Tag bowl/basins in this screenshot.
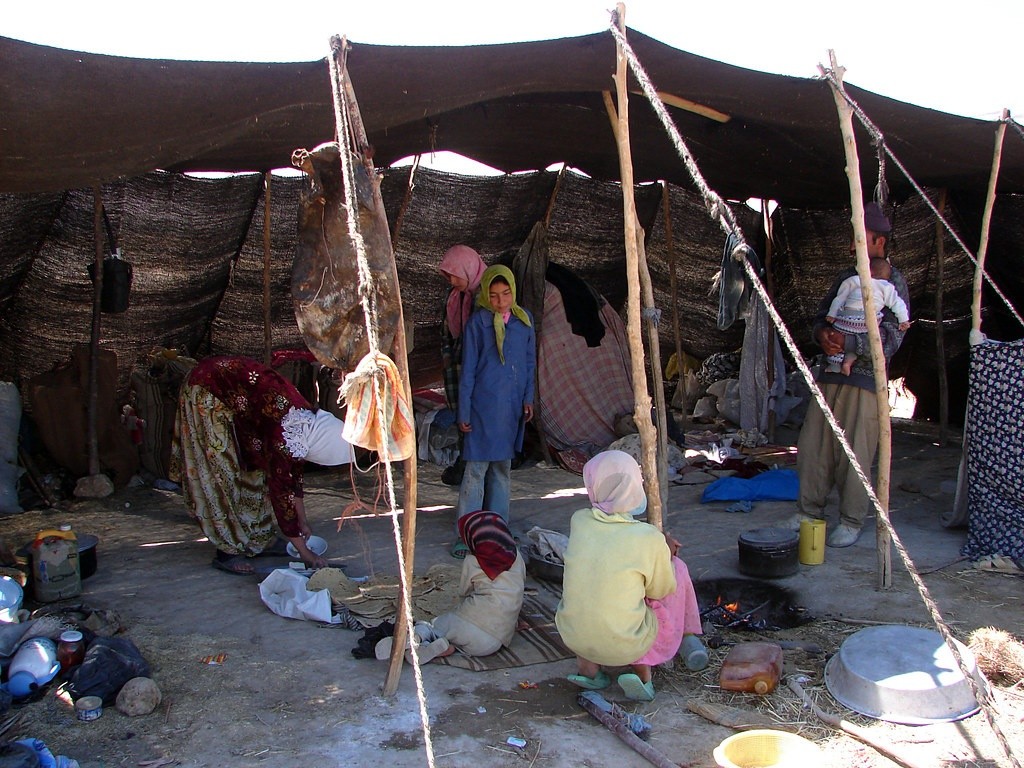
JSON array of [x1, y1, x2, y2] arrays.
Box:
[[824, 625, 991, 725]]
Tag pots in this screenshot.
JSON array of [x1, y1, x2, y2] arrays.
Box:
[[738, 528, 801, 578], [16, 532, 99, 581], [713, 730, 826, 768]]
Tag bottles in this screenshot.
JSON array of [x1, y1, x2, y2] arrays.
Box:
[[57, 631, 85, 682], [680, 634, 709, 671], [32, 525, 82, 601], [720, 642, 783, 694]]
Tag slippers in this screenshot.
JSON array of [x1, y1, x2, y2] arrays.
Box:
[[567, 670, 613, 690], [213, 550, 258, 577], [972, 552, 1024, 576], [617, 671, 656, 702], [449, 539, 471, 559], [258, 536, 290, 557]]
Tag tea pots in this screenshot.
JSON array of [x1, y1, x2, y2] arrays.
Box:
[[0, 637, 62, 696]]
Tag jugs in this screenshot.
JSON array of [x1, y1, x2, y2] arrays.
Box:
[[800, 519, 826, 564]]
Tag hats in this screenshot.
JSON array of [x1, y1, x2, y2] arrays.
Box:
[[862, 200, 892, 233]]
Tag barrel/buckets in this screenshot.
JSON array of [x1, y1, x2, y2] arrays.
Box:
[[0, 576, 23, 626]]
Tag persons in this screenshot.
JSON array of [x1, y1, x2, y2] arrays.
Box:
[[772, 200, 909, 548], [375, 510, 526, 667], [438, 245, 537, 559], [168, 355, 356, 578], [554, 449, 702, 701], [825, 258, 909, 376]]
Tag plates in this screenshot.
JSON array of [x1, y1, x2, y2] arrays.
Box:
[[287, 536, 328, 558]]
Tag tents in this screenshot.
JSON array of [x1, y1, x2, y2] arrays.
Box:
[[0, 0, 1024, 692]]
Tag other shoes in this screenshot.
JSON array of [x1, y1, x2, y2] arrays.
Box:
[[404, 636, 450, 667], [375, 631, 420, 661], [826, 523, 863, 548], [771, 513, 817, 534]]
